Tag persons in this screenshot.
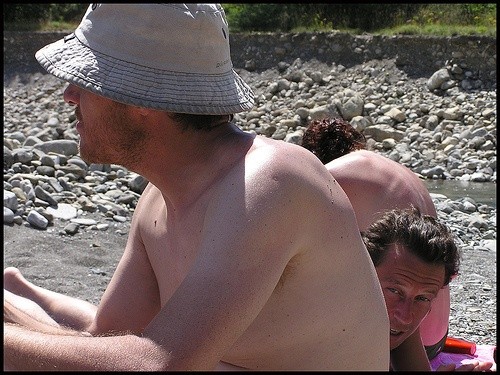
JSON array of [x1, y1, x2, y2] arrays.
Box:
[[301, 119, 450, 361], [3, 209, 493, 372], [3, 4, 390, 371]]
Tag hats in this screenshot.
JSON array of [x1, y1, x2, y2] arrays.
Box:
[[34, 4, 255, 115]]
